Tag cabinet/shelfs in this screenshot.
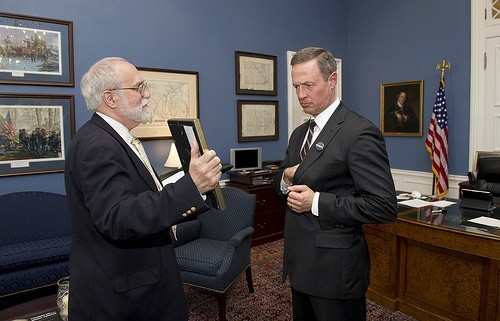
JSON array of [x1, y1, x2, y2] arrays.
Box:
[[223, 180, 284, 247]]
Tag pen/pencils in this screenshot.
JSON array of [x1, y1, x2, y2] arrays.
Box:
[[431, 210, 447, 213]]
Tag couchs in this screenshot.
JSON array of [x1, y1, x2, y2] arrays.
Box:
[[0, 191, 72, 301]]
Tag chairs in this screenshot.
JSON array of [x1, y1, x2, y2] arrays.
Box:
[[459, 154, 500, 209], [174, 187, 257, 321]]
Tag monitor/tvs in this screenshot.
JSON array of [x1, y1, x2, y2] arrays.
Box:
[[230, 147, 262, 173]]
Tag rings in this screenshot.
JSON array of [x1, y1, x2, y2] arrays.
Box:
[[291, 203, 293, 209]]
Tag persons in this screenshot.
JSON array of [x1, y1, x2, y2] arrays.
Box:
[[64, 57, 222, 321], [274, 46, 397, 321], [388, 89, 419, 133], [14, 43, 51, 62], [0, 127, 59, 156]]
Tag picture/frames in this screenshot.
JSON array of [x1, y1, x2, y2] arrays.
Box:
[[237, 100, 279, 143], [0, 93, 76, 175], [129, 67, 199, 140], [286, 50, 342, 143], [235, 50, 278, 96], [379, 80, 423, 137], [0, 12, 76, 87]]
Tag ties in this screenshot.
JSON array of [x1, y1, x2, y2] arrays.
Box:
[[131, 137, 177, 240], [301, 120, 315, 162]]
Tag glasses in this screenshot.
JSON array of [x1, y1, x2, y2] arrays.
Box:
[[109, 80, 148, 97]]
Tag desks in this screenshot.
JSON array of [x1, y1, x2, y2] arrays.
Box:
[[360, 192, 500, 321]]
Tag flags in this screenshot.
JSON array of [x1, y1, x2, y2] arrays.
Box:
[[425, 76, 449, 197], [3, 117, 14, 137], [424, 197, 446, 226]]
